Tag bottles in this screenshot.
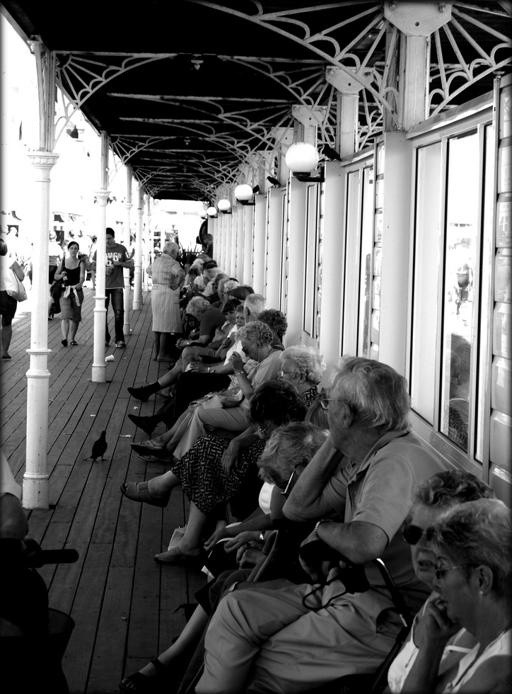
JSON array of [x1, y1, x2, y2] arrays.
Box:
[[62, 274, 69, 287]]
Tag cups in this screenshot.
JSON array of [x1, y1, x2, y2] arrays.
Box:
[[105, 355, 115, 362]]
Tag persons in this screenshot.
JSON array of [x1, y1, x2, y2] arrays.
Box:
[[50, 229, 97, 347], [369, 470, 512, 694], [176, 252, 254, 352], [92, 227, 134, 347], [0, 445, 29, 542], [146, 242, 185, 362], [195, 357, 446, 694], [0, 238, 24, 359], [119, 293, 329, 694]]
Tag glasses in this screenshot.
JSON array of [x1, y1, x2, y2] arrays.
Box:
[[401, 524, 437, 544]]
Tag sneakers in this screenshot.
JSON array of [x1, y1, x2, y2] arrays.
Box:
[[122, 384, 198, 694]]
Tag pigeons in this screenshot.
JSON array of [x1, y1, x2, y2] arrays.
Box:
[[90, 430, 108, 462]]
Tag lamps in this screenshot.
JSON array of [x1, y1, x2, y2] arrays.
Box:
[[285, 142, 325, 182], [235, 184, 255, 205], [207, 207, 218, 218], [218, 199, 232, 214]]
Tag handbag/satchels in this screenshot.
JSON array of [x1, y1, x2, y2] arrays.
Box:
[[4, 269, 27, 301], [50, 278, 62, 299]]
